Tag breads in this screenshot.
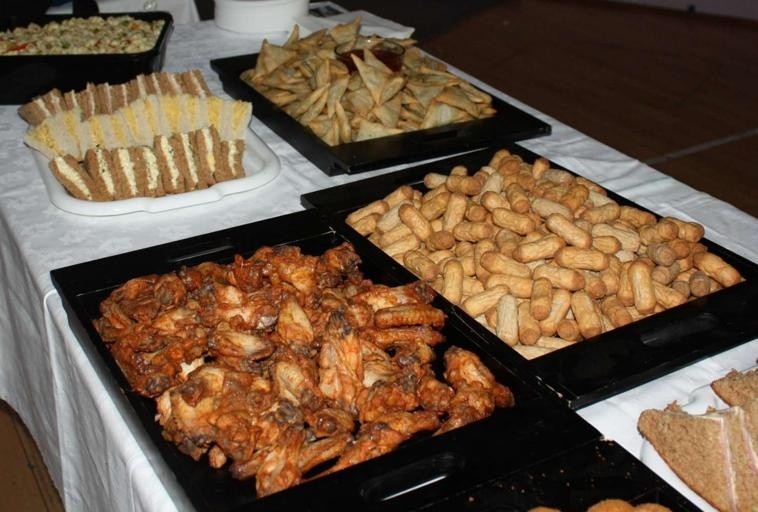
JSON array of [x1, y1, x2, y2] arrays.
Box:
[[17, 68, 253, 201], [638, 368, 758, 511]]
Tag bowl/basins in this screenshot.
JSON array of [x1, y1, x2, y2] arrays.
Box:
[[334, 41, 406, 74]]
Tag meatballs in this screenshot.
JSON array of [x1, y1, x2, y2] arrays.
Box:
[[346, 149, 741, 359]]
